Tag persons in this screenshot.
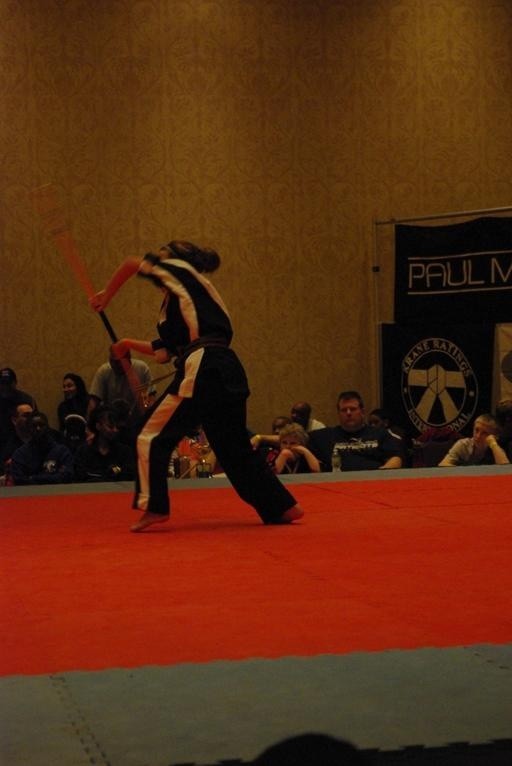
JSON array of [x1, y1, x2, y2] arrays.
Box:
[[267, 422, 330, 474], [84, 339, 161, 425], [306, 390, 405, 474], [244, 401, 326, 453], [433, 412, 512, 468], [89, 237, 307, 532], [255, 415, 290, 471], [1, 366, 221, 489], [365, 407, 408, 440]]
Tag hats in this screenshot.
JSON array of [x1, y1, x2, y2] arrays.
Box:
[[0, 368, 16, 383]]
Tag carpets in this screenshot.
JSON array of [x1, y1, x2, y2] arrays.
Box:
[[0, 475, 511, 685]]
